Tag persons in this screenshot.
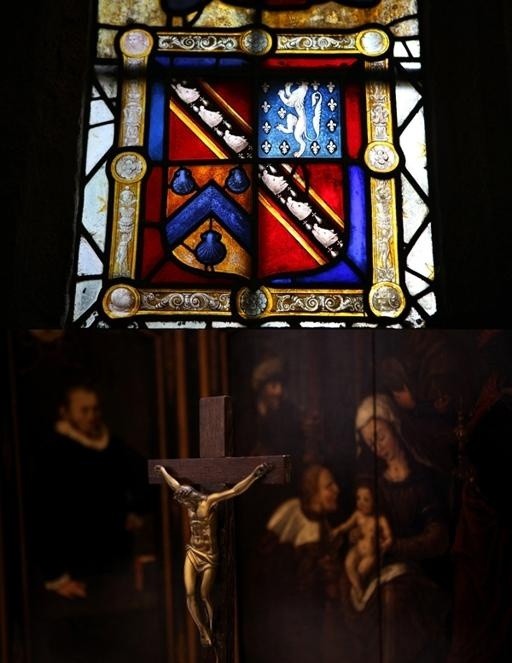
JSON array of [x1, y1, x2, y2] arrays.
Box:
[[231, 346, 323, 603], [345, 389, 454, 663], [254, 463, 345, 660], [11, 380, 164, 660], [328, 480, 394, 614], [153, 458, 274, 653], [392, 329, 510, 542]]
